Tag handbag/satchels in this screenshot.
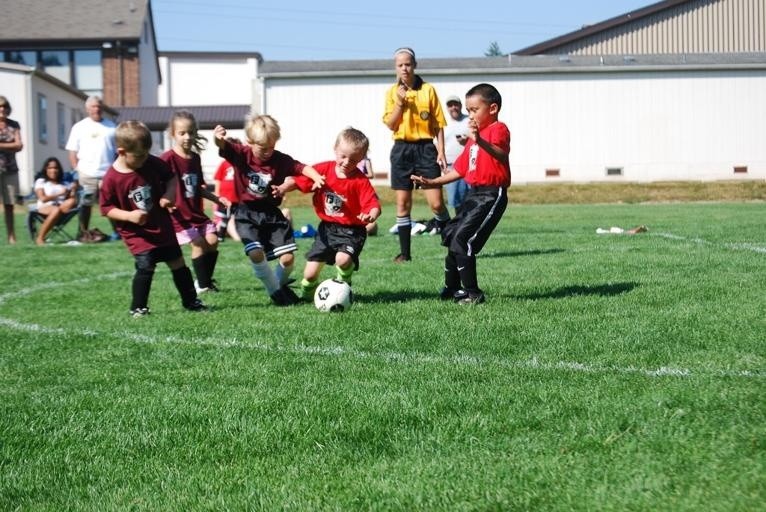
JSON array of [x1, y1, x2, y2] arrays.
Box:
[[78, 227, 106, 243]]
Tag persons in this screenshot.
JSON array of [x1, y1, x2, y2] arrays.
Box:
[[382, 46, 455, 262], [215, 115, 326, 305], [272, 128, 380, 302], [33, 156, 80, 246], [438, 94, 472, 216], [0, 95, 24, 244], [214, 160, 240, 241], [408, 83, 512, 305], [101, 117, 207, 317], [64, 95, 117, 242], [158, 113, 219, 296]]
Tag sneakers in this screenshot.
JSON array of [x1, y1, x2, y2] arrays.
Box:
[[440, 286, 464, 300], [282, 286, 300, 303], [455, 288, 485, 306], [394, 254, 411, 263], [129, 306, 150, 319], [195, 284, 219, 296], [184, 299, 206, 312]]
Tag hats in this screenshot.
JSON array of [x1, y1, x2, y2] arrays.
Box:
[[445, 95, 462, 105]]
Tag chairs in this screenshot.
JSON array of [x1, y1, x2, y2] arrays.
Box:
[[25, 171, 87, 245]]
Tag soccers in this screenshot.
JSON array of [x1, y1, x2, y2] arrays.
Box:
[[314, 279, 353, 313]]
